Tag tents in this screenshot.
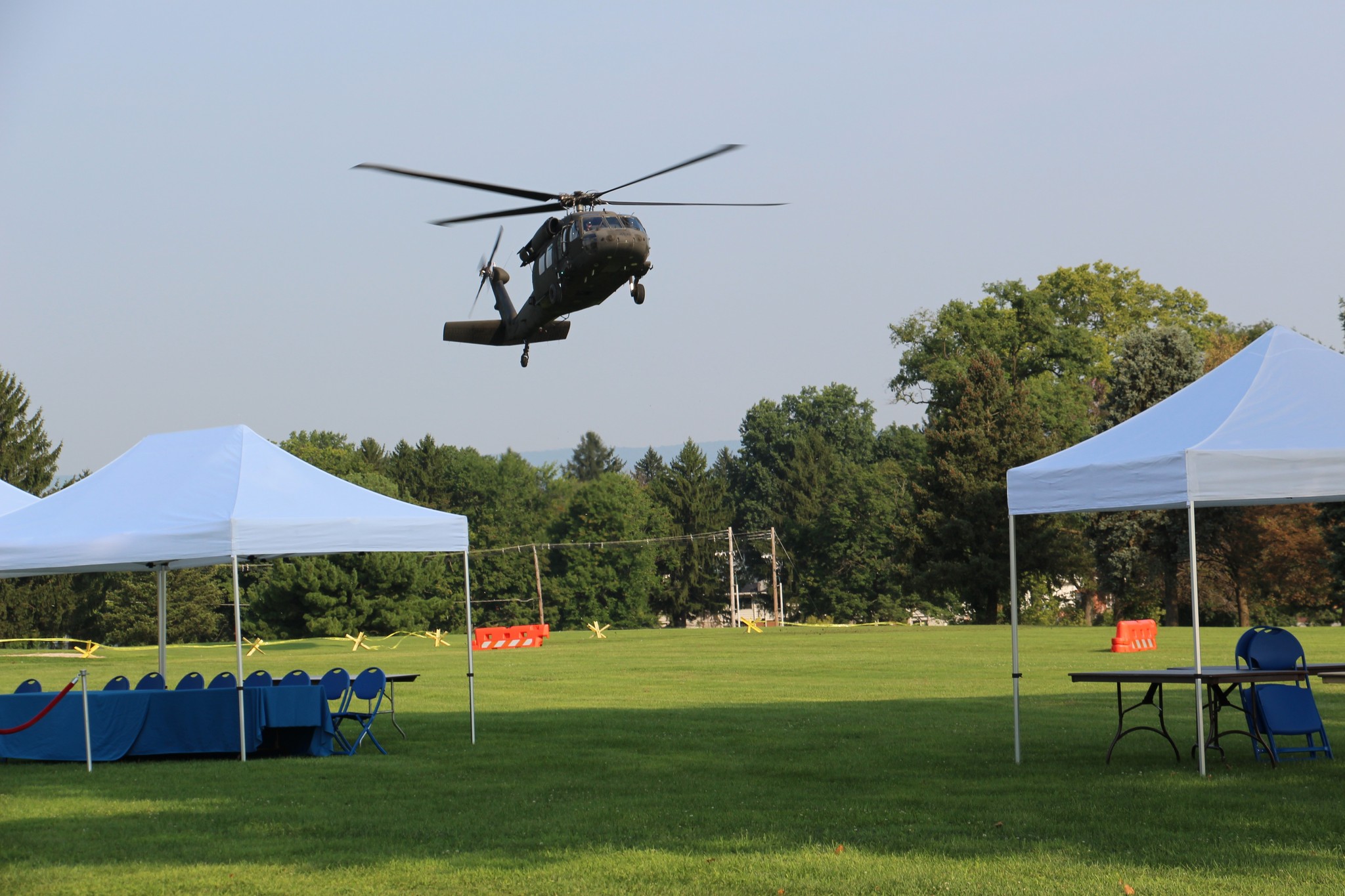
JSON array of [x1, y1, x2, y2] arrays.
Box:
[[1005, 326, 1345, 778], [0, 423, 477, 761]]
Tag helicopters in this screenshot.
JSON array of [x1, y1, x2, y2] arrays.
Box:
[[353, 139, 796, 369]]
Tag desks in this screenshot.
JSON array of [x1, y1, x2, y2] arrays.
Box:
[[0, 685, 333, 761], [1168, 664, 1345, 763], [1067, 670, 1310, 771], [243, 674, 421, 747]]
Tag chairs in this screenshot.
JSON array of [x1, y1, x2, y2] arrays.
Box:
[[276, 670, 312, 686], [134, 672, 165, 690], [318, 667, 351, 754], [1235, 625, 1319, 762], [327, 666, 388, 755], [13, 679, 42, 694], [207, 672, 237, 689], [175, 672, 204, 690], [103, 675, 130, 690], [1247, 627, 1336, 761], [243, 670, 273, 687]]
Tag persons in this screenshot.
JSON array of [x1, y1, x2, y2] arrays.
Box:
[[583, 220, 593, 233], [626, 220, 636, 230]]
